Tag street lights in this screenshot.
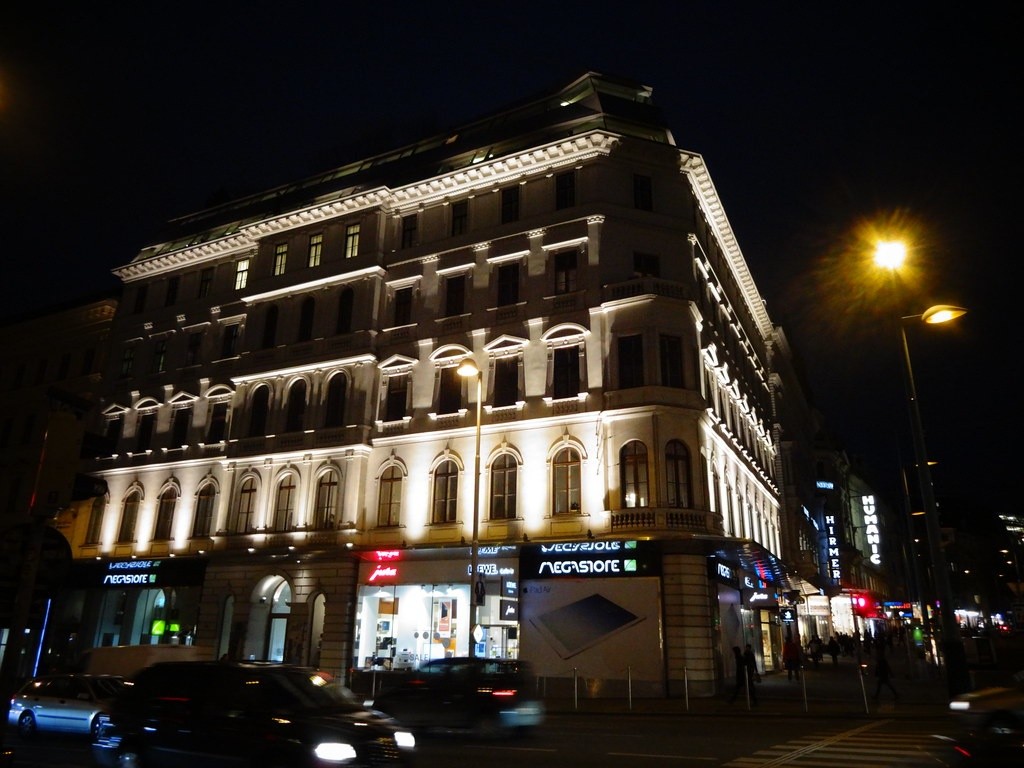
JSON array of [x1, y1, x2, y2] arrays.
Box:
[[897, 302, 972, 687], [455, 357, 485, 658], [898, 457, 939, 679]]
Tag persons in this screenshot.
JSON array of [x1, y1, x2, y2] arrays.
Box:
[[806, 622, 912, 681], [741, 643, 758, 696], [874, 655, 895, 697], [783, 637, 804, 684]]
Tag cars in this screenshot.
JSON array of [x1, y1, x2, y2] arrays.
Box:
[[6, 673, 133, 742], [365, 657, 537, 744], [108, 659, 417, 768]]
